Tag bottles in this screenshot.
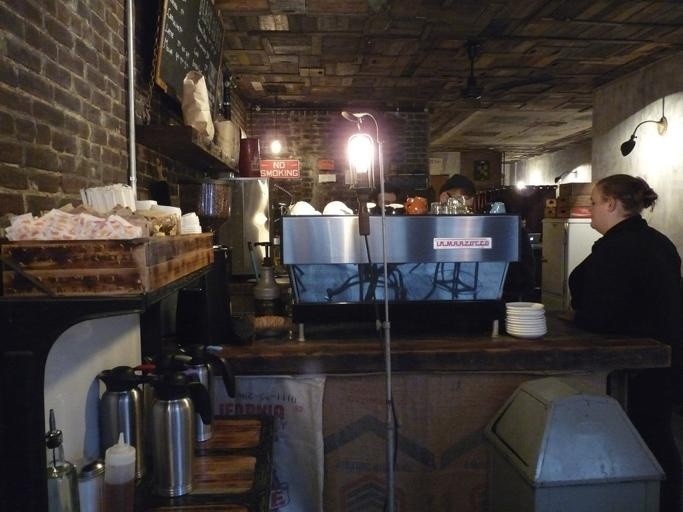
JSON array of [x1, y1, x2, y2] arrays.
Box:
[[45, 408, 137, 510], [254, 256, 281, 316]]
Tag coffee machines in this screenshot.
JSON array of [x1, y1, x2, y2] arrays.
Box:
[[181, 179, 232, 320]]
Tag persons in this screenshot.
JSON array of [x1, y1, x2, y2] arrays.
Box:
[[489, 197, 538, 304], [369, 183, 398, 216], [564, 172, 683, 512]]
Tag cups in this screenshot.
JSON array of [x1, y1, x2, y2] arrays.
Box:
[[239, 138, 261, 177], [432, 195, 466, 215]]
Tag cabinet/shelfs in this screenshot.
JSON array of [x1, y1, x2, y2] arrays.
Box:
[[0, 232, 274, 512]]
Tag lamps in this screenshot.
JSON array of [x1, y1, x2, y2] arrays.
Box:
[[555, 170, 578, 183], [460, 43, 484, 105], [342, 111, 398, 512], [621, 115, 667, 156], [268, 89, 282, 153]]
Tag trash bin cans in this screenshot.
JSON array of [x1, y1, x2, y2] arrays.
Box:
[[480, 375, 668, 512]]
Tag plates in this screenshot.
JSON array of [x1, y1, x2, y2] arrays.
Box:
[[504, 301, 548, 339]]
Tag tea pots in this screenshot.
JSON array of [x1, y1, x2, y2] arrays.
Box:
[[95, 340, 236, 499]]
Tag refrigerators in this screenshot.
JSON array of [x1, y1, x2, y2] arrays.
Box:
[[539, 215, 615, 316]]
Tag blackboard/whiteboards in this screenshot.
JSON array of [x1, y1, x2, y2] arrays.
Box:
[[224, 72, 248, 135], [153, 0, 226, 121]]
